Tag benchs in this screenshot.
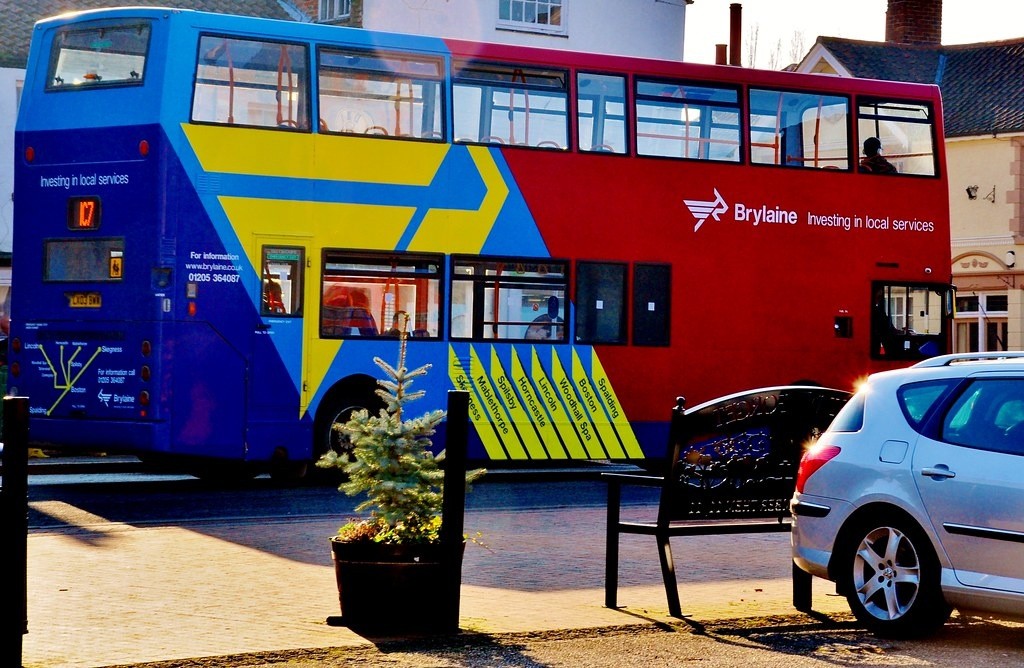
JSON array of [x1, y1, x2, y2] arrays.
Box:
[[600, 385, 855, 616]]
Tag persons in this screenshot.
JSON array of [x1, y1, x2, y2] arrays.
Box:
[[871, 280, 905, 347], [523, 295, 565, 340], [379, 311, 416, 338], [0, 286, 10, 336], [861, 136, 898, 175], [263, 283, 285, 314]]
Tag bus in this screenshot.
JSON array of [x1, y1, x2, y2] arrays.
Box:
[[7, 6, 955, 489]]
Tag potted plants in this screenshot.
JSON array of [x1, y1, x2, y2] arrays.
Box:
[[315, 313, 495, 639]]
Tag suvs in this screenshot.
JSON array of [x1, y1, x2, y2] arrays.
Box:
[[788, 351, 1024, 638]]
